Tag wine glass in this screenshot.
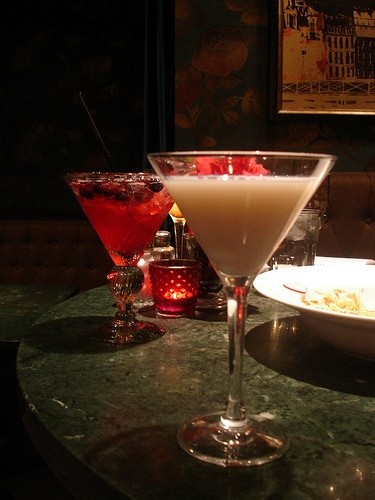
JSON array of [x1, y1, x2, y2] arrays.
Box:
[[61, 171, 180, 347], [184, 232, 229, 309], [147, 150, 339, 468], [166, 197, 189, 259]]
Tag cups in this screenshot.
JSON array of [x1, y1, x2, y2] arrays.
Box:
[[261, 173, 331, 267]]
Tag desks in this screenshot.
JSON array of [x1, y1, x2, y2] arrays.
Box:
[[18, 283, 375, 496], [0, 283, 79, 343]]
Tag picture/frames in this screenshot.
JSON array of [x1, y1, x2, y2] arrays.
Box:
[[277, 0, 374, 115]]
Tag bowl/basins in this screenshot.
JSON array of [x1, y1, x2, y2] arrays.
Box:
[[252, 264, 375, 361]]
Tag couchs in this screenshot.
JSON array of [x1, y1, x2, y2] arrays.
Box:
[[316, 171, 375, 259], [1, 218, 104, 281]]
